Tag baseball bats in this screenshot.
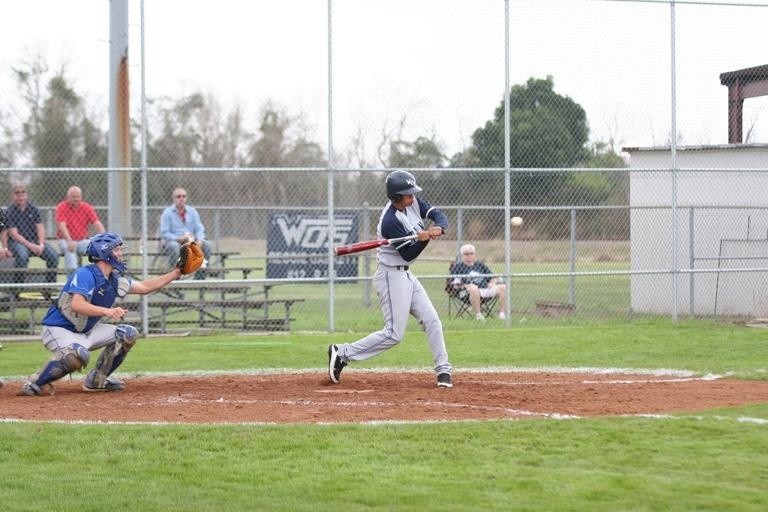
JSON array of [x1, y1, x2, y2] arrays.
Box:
[[335, 228, 445, 256]]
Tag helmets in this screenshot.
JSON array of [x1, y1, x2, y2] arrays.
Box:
[[86, 233, 128, 272], [386, 171, 422, 195]]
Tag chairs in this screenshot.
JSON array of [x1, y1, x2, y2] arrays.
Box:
[[444, 264, 499, 322]]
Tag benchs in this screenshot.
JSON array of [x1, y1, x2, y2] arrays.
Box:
[[0, 234, 307, 336]]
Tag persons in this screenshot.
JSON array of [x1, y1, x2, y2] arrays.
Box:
[[160, 188, 213, 281], [55, 185, 107, 277], [4, 183, 59, 302], [22, 231, 204, 396], [0, 207, 15, 302], [450, 244, 507, 322], [329, 169, 454, 387]]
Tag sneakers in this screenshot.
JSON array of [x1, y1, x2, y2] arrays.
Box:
[[22, 382, 36, 396], [437, 373, 453, 388], [327, 343, 347, 384], [82, 380, 124, 392]]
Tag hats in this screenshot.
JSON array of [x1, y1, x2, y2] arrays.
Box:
[[12, 184, 27, 193]]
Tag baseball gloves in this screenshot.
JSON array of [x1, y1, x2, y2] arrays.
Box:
[[176, 241, 204, 275]]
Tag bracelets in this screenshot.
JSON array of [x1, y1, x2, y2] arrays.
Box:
[[5, 248, 8, 250]]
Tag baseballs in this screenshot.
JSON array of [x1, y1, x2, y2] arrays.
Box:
[[511, 217, 523, 226]]
